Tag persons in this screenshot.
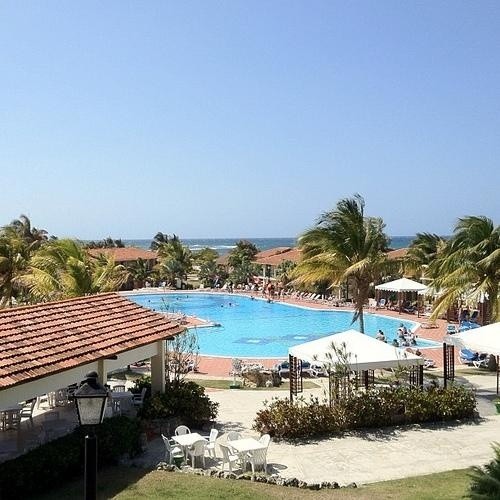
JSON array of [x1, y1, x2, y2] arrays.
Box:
[[221, 300, 232, 307], [262, 279, 285, 298], [146, 295, 191, 302], [375, 323, 421, 356]]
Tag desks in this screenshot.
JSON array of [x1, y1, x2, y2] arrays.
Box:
[[110, 390, 134, 412], [1, 404, 22, 427]]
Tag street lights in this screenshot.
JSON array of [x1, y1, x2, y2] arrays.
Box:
[[268, 266, 271, 284], [68, 372, 109, 500]]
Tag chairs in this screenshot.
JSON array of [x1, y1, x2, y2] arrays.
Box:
[[162, 425, 271, 475], [275, 361, 310, 378], [114, 384, 147, 417], [20, 399, 36, 426], [292, 291, 321, 303], [380, 299, 403, 311]]
